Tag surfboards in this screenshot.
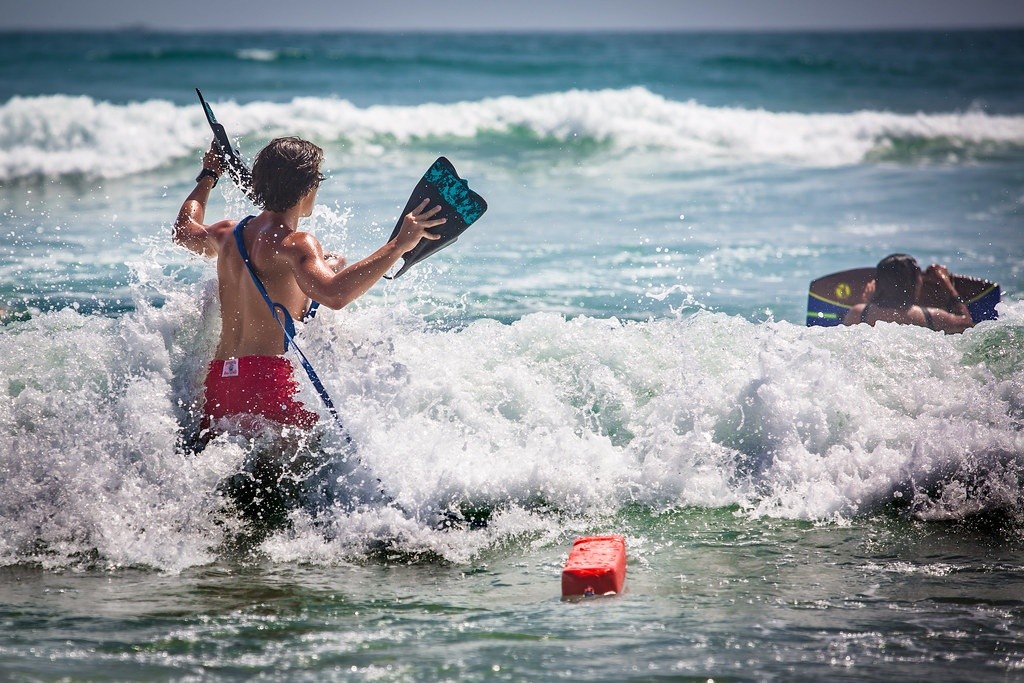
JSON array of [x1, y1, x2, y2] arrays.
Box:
[[806, 267, 1001, 333]]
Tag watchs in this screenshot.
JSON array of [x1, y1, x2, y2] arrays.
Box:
[[195, 168, 219, 189]]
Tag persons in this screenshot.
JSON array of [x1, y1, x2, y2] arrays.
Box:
[[843, 253, 973, 336], [171, 136, 446, 462]]
